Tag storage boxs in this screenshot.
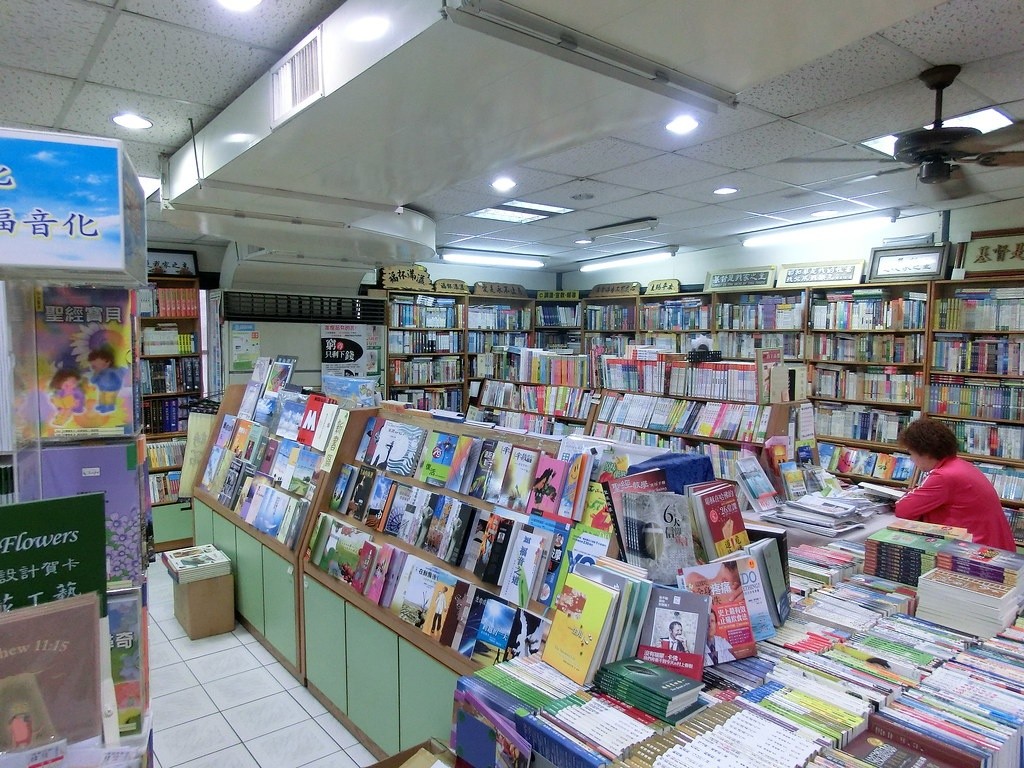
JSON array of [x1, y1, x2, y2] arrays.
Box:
[[173, 576, 234, 640], [360, 737, 476, 768]]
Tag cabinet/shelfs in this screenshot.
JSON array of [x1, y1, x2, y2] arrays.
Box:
[[139, 278, 1024, 768]]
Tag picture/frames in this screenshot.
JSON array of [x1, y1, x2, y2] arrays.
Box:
[[951, 227, 1024, 276], [865, 241, 951, 279], [148, 246, 201, 277], [703, 264, 777, 292], [776, 259, 864, 287]]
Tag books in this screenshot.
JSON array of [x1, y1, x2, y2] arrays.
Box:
[[0, 281, 1024, 768]]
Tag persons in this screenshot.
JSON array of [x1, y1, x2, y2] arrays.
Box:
[[895, 419, 1017, 554]]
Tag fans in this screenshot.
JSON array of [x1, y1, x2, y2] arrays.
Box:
[[776, 64, 1024, 199]]
